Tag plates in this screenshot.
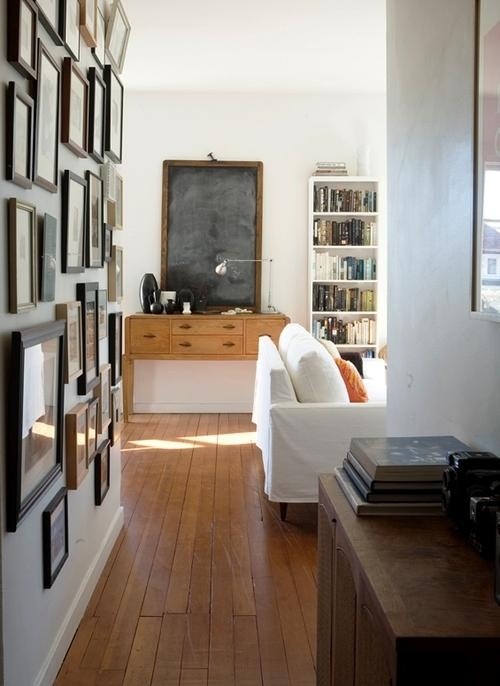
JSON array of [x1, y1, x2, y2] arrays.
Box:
[[221, 312, 236, 316]]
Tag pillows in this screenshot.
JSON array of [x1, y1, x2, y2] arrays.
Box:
[[318, 337, 342, 360], [337, 358, 371, 402]]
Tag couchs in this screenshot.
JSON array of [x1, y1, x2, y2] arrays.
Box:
[[252, 336, 388, 524]]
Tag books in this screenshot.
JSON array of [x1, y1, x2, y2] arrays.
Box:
[[314, 158, 349, 176], [313, 184, 377, 377], [332, 433, 478, 520]]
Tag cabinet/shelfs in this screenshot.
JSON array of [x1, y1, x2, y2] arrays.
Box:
[[313, 469, 497, 683], [122, 311, 291, 422], [308, 176, 380, 351]]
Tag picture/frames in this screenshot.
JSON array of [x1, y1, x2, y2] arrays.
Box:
[[34, 2, 65, 46], [58, 2, 80, 63], [41, 487, 69, 589], [87, 66, 107, 164], [102, 64, 124, 166], [60, 57, 88, 160], [83, 399, 99, 465], [110, 388, 121, 446], [95, 289, 109, 375], [40, 212, 58, 302], [61, 169, 88, 274], [92, 363, 112, 435], [100, 161, 124, 232], [7, 197, 39, 316], [65, 402, 88, 492], [7, 321, 63, 532], [79, 2, 97, 48], [75, 282, 102, 397], [106, 1, 132, 73], [92, 7, 106, 69], [94, 440, 111, 506], [28, 38, 61, 197], [84, 170, 104, 269], [102, 221, 113, 262], [6, 2, 38, 79], [4, 81, 35, 190], [106, 245, 123, 303], [56, 300, 83, 384]]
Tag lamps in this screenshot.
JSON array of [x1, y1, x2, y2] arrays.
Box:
[[214, 257, 280, 314]]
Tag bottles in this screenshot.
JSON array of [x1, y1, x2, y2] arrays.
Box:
[[165, 299, 176, 314], [325, 290, 334, 311], [149, 288, 164, 313]]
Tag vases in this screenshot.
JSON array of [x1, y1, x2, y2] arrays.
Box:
[[139, 272, 196, 314]]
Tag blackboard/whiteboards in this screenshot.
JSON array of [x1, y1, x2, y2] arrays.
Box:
[[160, 160, 263, 313]]
